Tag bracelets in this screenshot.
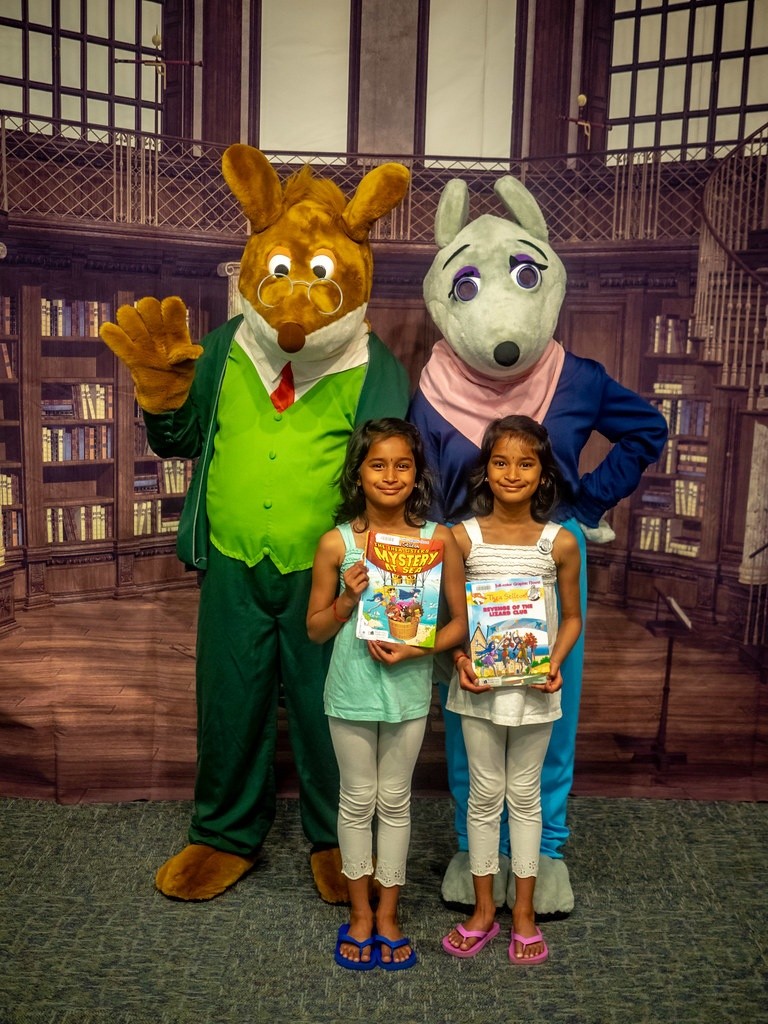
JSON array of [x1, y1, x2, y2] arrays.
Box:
[[334, 597, 349, 622], [455, 654, 470, 670]]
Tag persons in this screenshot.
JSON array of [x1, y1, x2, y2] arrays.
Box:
[[440, 415, 577, 963], [307, 417, 467, 967]]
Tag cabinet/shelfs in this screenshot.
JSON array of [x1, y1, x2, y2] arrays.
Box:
[[0, 213, 715, 636]]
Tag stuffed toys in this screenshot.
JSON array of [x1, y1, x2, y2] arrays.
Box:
[[405, 172, 667, 914], [100, 144, 413, 903]]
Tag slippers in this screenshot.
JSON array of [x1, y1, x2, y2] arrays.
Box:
[[375, 933, 417, 971], [508, 924, 549, 964], [441, 921, 501, 958], [333, 922, 376, 970]]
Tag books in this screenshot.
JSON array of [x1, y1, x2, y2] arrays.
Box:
[[356, 530, 444, 648], [464, 575, 551, 687], [0, 297, 710, 568]]
